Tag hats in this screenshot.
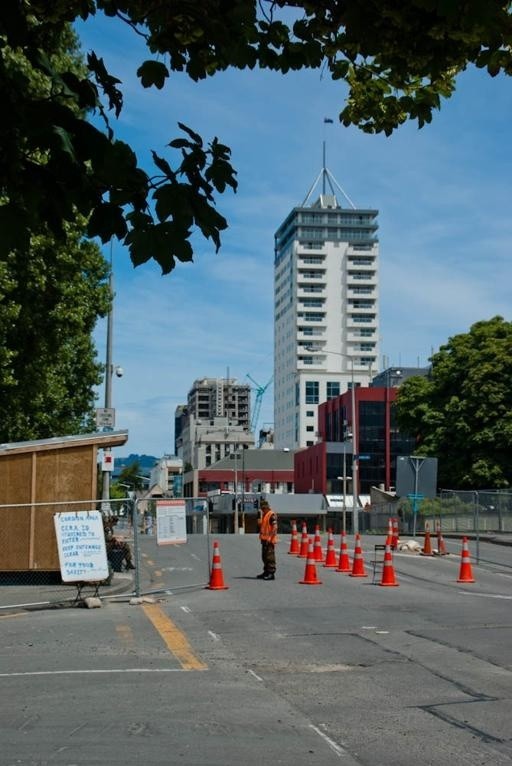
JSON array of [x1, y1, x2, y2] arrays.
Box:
[[258, 500, 271, 509]]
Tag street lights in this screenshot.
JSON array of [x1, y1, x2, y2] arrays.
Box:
[[382, 367, 403, 491], [336, 476, 353, 533], [237, 441, 250, 536], [303, 345, 362, 536]]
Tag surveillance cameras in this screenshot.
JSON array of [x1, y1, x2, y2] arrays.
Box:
[[115, 367, 124, 377]]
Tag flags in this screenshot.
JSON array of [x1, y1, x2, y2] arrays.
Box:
[[324, 117, 334, 124]]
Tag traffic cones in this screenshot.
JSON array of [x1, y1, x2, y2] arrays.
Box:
[[203, 539, 230, 591], [454, 535, 478, 584], [286, 516, 450, 587]]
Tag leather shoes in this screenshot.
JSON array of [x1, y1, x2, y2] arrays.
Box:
[[257, 572, 274, 580]]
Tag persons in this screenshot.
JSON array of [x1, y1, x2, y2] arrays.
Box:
[[254, 501, 279, 581], [103, 515, 136, 571]]
